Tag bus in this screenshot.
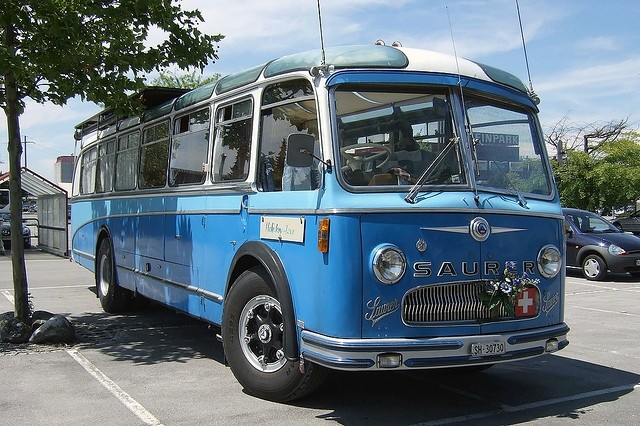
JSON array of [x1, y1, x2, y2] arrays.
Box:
[[0, 188, 11, 220], [70, 39, 570, 404]]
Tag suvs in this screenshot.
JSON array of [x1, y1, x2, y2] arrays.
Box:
[[0, 220, 32, 250]]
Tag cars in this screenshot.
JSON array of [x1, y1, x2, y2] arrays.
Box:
[[561, 208, 640, 281], [613, 210, 640, 236]]
